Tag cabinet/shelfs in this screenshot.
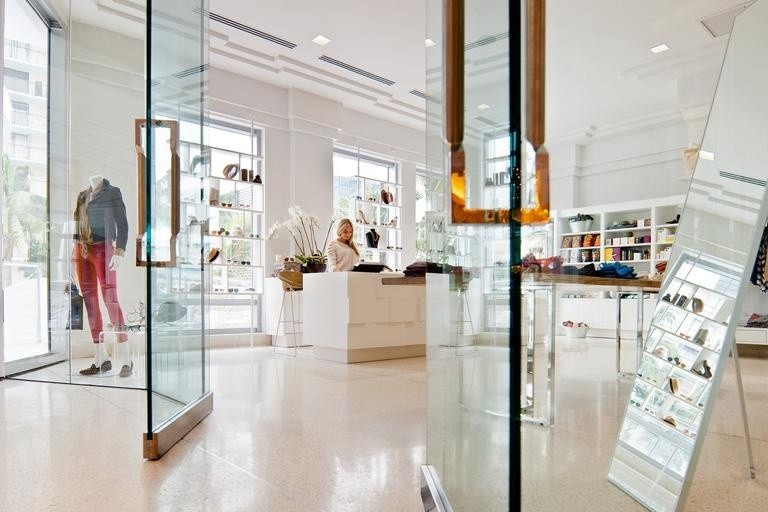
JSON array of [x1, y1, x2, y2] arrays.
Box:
[[428, 228, 469, 258], [631, 273, 739, 445], [350, 172, 409, 271], [160, 138, 267, 298], [555, 194, 688, 299], [733, 328, 768, 346]]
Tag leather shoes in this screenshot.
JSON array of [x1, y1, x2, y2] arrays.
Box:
[[118, 360, 134, 377], [703, 359, 712, 378], [79, 361, 112, 375]]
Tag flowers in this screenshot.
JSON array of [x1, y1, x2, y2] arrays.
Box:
[[265, 201, 343, 269]]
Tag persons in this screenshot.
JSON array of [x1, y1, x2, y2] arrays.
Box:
[[325, 219, 359, 272], [73, 177, 133, 376]]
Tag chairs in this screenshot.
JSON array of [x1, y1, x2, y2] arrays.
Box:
[[446, 268, 479, 360], [271, 269, 307, 359]]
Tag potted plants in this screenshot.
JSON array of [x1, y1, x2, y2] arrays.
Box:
[[566, 211, 595, 235]]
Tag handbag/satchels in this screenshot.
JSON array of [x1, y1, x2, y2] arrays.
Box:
[[278, 271, 303, 291], [63, 282, 84, 330]]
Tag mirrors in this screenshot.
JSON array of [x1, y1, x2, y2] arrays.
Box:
[[600, 1, 768, 512]]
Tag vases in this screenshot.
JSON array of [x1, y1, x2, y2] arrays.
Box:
[[300, 262, 327, 272]]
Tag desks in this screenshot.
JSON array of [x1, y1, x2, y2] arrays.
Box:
[[485, 262, 662, 426]]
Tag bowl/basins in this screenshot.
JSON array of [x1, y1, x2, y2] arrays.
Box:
[[563, 325, 591, 339], [568, 218, 594, 233]]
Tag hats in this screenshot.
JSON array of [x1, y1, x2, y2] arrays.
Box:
[[205, 247, 222, 263], [223, 163, 240, 180], [661, 414, 678, 428], [381, 189, 393, 204], [154, 301, 188, 322], [692, 297, 704, 314], [669, 376, 680, 395]]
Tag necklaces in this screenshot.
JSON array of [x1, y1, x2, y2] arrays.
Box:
[[370, 231, 378, 242]]
